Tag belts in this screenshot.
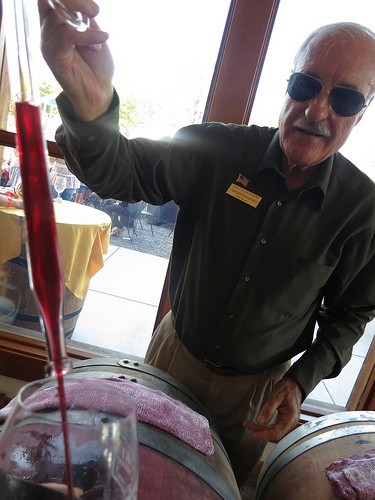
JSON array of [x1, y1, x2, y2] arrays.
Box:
[[170, 313, 254, 376]]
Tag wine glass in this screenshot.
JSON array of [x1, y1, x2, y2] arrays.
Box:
[[0, 377, 137, 500], [54, 175, 67, 202]]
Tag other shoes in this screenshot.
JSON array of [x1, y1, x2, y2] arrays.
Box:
[[110, 226, 125, 236]]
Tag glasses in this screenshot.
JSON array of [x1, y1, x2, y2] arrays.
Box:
[[286, 72, 368, 117]]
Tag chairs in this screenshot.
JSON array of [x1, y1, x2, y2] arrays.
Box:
[[111, 211, 154, 240]]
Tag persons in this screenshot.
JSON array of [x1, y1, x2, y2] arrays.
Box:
[[0, 148, 21, 188], [0, 195, 24, 209], [49, 157, 147, 235], [33, 0, 375, 491]]
